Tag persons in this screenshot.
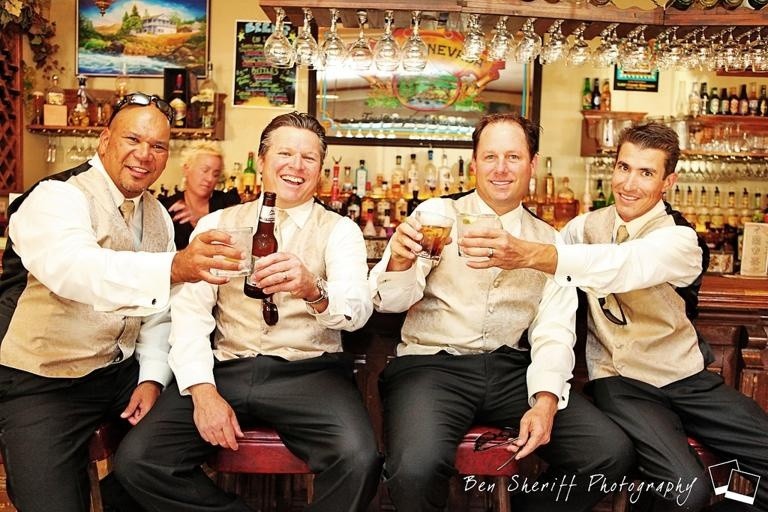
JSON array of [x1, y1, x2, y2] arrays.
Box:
[[111, 110, 386, 512], [366, 112, 635, 512], [0, 93, 247, 512], [157, 138, 240, 250], [458, 123, 768, 510]]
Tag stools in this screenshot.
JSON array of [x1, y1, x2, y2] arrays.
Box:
[[83, 418, 116, 512], [212, 429, 314, 510], [687, 439, 724, 511], [449, 425, 521, 511]]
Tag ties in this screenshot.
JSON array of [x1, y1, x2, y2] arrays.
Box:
[[614, 225, 628, 244], [120, 199, 134, 226], [273, 210, 287, 252]]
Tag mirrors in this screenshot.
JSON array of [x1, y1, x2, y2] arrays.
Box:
[[310, 23, 543, 149]]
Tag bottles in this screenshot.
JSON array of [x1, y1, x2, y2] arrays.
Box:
[[212, 149, 575, 203], [46, 75, 66, 105], [690, 80, 767, 118], [596, 118, 687, 151], [319, 185, 423, 237], [527, 174, 580, 227], [736, 187, 753, 264], [583, 77, 613, 112], [67, 61, 217, 128], [594, 179, 768, 251], [243, 189, 280, 300]]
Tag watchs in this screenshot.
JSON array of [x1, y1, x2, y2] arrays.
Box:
[[303, 274, 328, 304]]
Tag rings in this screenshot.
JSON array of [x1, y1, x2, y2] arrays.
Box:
[[487, 248, 494, 259], [282, 272, 287, 283]]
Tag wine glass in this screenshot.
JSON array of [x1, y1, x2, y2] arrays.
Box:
[[688, 121, 743, 152], [262, 6, 768, 75], [592, 156, 768, 185], [335, 119, 474, 141], [33, 92, 44, 125], [45, 134, 205, 165]]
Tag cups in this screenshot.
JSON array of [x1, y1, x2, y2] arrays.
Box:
[[457, 210, 494, 262], [414, 209, 455, 264], [750, 134, 767, 148], [215, 222, 252, 279]]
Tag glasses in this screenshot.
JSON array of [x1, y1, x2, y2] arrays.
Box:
[[262, 294, 279, 326], [108, 91, 174, 125], [472, 426, 524, 471], [598, 294, 627, 326]]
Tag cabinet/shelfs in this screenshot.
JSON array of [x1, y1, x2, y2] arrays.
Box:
[[234, 192, 579, 264], [26, 86, 227, 141], [579, 109, 767, 161]]
